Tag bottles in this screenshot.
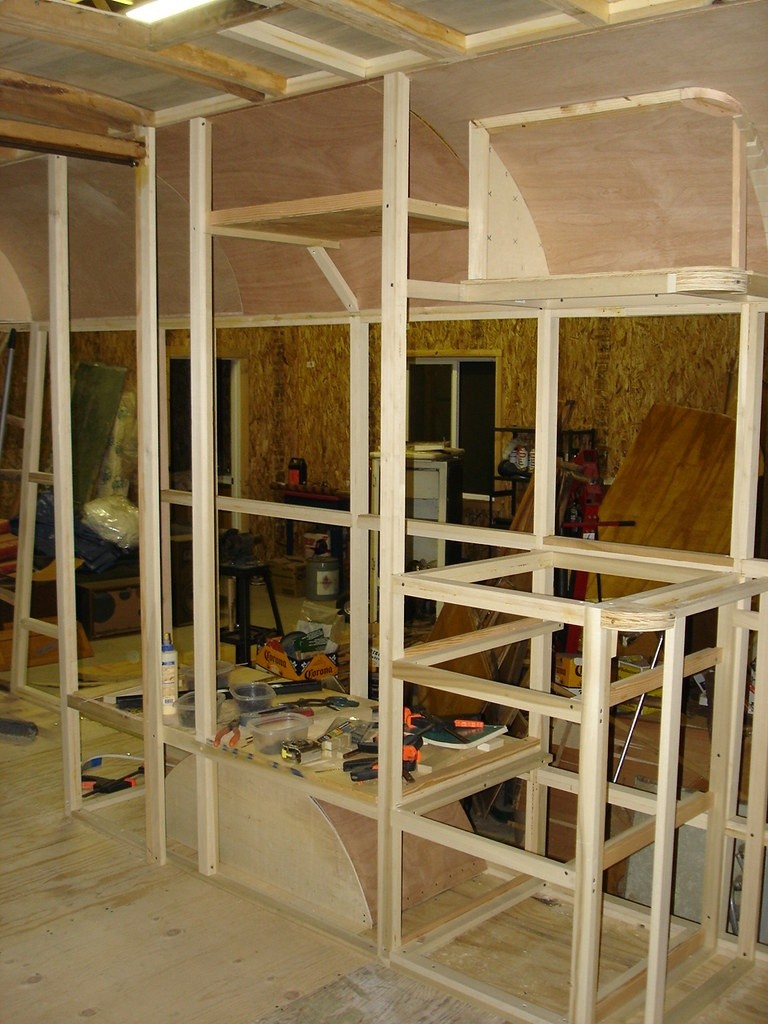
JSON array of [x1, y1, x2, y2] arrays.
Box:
[[510, 450, 517, 463], [519, 448, 528, 468], [161, 633, 178, 715], [530, 449, 535, 470]]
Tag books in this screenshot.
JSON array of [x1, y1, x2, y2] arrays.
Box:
[[421, 717, 508, 749]]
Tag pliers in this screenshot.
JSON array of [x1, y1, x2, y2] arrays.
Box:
[[213, 718, 242, 750], [340, 756, 378, 782]]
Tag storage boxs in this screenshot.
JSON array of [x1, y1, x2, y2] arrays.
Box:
[[553, 651, 583, 689], [76, 584, 141, 642], [616, 654, 665, 716], [268, 553, 308, 599]]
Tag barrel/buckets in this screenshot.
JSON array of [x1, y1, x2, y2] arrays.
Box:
[[307, 557, 340, 601]]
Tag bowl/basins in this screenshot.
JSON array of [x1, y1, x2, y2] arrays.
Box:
[[182, 660, 235, 690], [173, 692, 225, 726], [247, 713, 314, 755], [229, 682, 276, 713]]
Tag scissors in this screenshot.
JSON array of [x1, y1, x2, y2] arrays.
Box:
[[277, 695, 359, 711]]
